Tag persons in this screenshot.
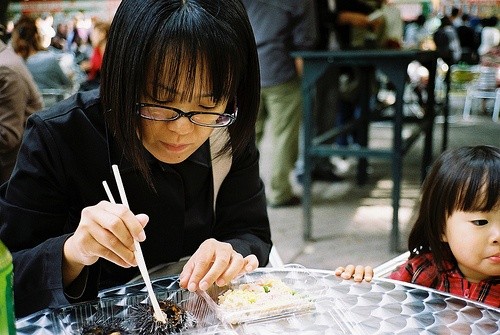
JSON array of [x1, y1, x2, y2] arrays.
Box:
[[243, 0, 317, 208], [0, 0, 273, 321], [335, 145, 500, 307], [404, 7, 500, 65], [0, 39, 44, 175], [293, 0, 403, 182], [8, 13, 112, 106]]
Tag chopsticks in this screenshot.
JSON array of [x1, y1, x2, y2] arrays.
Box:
[[102, 164, 166, 324]]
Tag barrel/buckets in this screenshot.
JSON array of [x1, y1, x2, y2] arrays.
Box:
[[477, 70, 495, 91]]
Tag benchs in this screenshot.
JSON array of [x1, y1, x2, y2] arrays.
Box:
[[446, 63, 500, 122]]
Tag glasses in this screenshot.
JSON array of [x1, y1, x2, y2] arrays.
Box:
[[135, 82, 238, 127]]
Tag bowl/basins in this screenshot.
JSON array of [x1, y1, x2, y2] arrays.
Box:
[[198, 263, 370, 335], [51, 287, 223, 335]]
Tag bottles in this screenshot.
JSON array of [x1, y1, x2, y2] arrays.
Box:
[[0, 240, 18, 335], [449, 60, 476, 92]]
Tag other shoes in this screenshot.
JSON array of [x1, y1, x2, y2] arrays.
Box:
[[274, 196, 300, 207], [312, 171, 344, 182]]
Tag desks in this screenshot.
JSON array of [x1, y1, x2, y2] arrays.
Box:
[[290, 48, 452, 252], [13, 264, 500, 335]]
